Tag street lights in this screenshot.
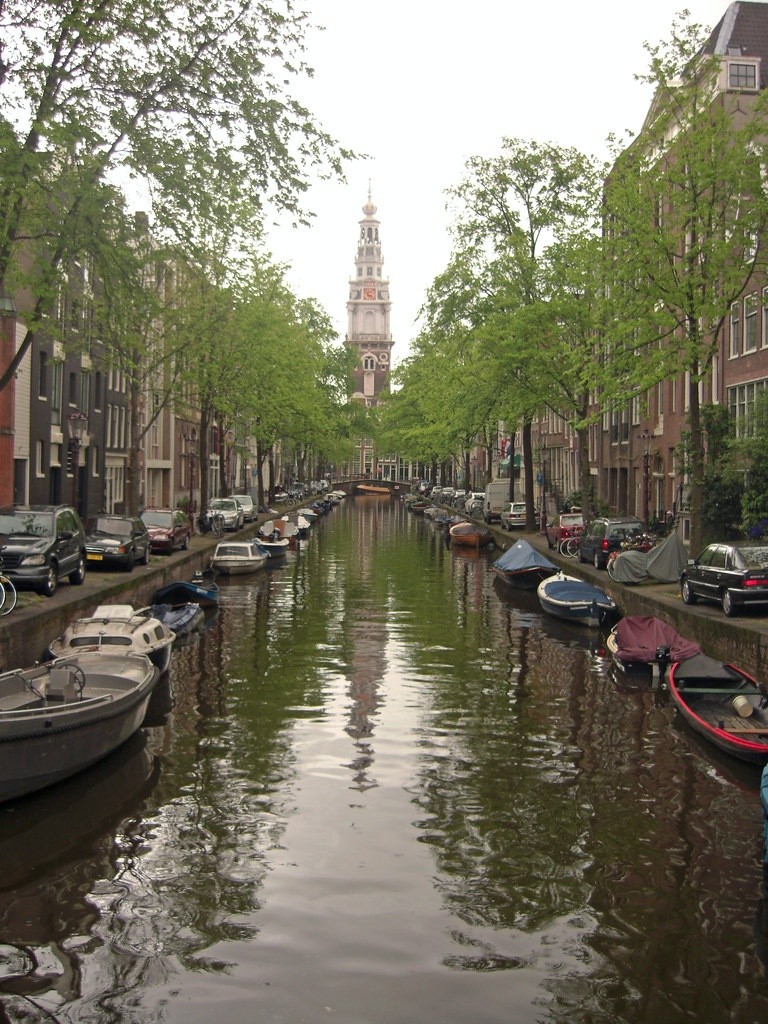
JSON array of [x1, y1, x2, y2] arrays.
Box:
[[471, 455, 477, 491], [635, 428, 655, 533], [538, 443, 551, 537], [241, 446, 251, 494], [64, 406, 90, 509], [184, 437, 200, 538]]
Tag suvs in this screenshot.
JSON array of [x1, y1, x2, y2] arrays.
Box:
[[0, 502, 87, 598], [577, 517, 648, 570]]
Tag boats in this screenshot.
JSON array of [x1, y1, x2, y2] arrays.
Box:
[[0, 646, 160, 803], [154, 570, 218, 606], [491, 540, 560, 590], [252, 488, 348, 557], [0, 730, 164, 893], [397, 492, 465, 531], [607, 617, 701, 681], [537, 570, 617, 628], [134, 600, 204, 640], [47, 604, 177, 678], [209, 540, 269, 575], [449, 520, 492, 549], [668, 645, 768, 768]]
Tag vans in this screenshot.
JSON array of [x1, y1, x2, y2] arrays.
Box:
[[480, 477, 522, 525]]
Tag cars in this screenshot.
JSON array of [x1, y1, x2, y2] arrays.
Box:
[[410, 477, 486, 515], [81, 514, 151, 574], [544, 514, 596, 553], [140, 507, 191, 556], [499, 503, 540, 531], [680, 540, 768, 616], [205, 497, 245, 532], [273, 479, 329, 508], [227, 494, 258, 522]]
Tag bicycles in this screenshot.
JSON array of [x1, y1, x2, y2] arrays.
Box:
[[196, 510, 225, 537], [559, 525, 584, 559], [606, 531, 659, 584], [0, 556, 20, 617]]
